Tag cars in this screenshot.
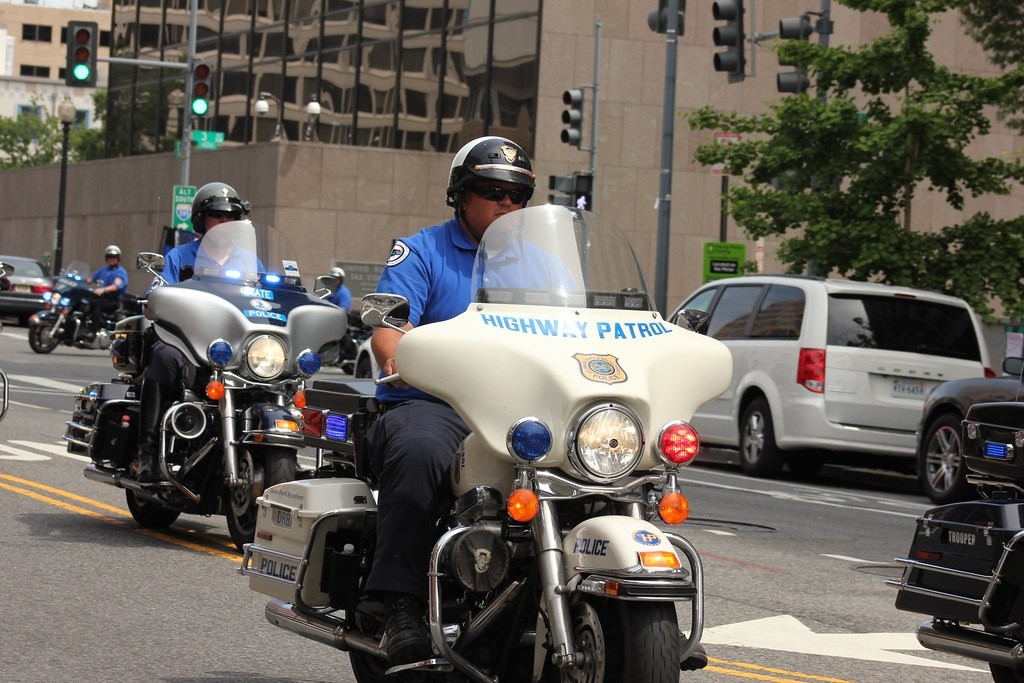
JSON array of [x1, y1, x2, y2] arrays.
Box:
[[916, 356, 1024, 505], [0, 254, 54, 328]]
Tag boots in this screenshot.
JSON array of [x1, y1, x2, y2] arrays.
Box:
[[137, 378, 167, 483]]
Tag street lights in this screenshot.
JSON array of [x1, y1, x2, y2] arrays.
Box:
[[53, 93, 76, 275]]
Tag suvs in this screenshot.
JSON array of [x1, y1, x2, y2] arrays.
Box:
[[667, 274, 996, 481]]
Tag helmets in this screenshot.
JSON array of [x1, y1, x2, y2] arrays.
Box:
[[446, 135, 536, 207], [328, 267, 345, 278], [105, 244, 122, 256], [190, 183, 244, 232]]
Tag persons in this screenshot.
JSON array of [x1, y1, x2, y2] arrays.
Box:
[[322, 267, 351, 313], [363, 136, 577, 666], [85, 244, 129, 332], [137, 181, 265, 484]]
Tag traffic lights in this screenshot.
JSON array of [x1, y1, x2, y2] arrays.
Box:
[[560, 87, 583, 146], [776, 15, 811, 94], [190, 60, 212, 118], [548, 170, 594, 212], [711, 0, 747, 84], [65, 21, 98, 89]]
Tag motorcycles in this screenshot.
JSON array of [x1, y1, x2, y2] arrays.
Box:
[[883, 401, 1024, 683], [28, 259, 139, 354], [237, 200, 737, 683], [61, 218, 350, 553]]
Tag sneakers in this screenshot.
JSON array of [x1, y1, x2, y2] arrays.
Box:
[[384, 602, 429, 662]]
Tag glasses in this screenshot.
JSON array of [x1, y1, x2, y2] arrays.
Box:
[[474, 185, 526, 205], [209, 210, 236, 218]]
